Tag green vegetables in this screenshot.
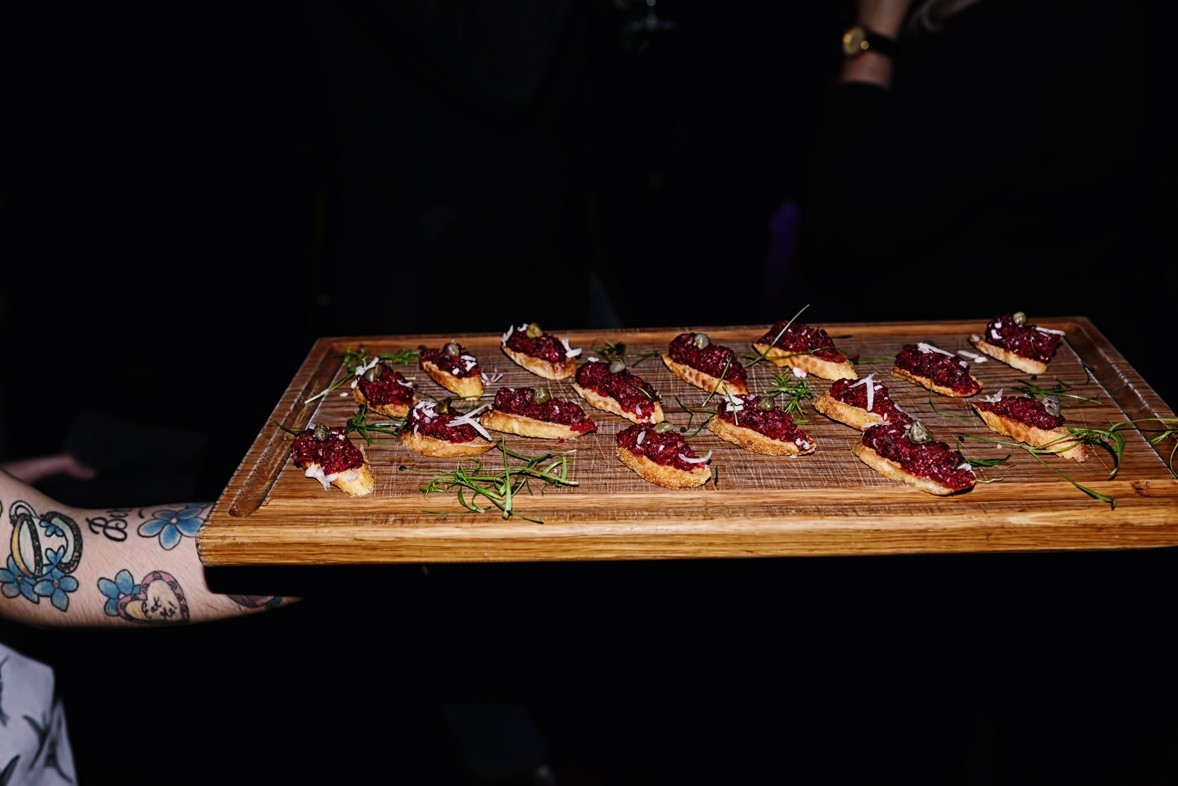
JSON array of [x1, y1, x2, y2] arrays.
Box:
[[263, 344, 1178, 524]]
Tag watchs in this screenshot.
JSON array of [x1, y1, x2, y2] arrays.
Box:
[[842, 22, 898, 61]]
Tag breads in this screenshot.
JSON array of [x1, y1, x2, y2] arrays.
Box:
[[293, 324, 1089, 495]]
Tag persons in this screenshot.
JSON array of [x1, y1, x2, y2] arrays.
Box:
[[0, 429, 95, 484], [0, 468, 305, 625], [796, 0, 1141, 318]]
